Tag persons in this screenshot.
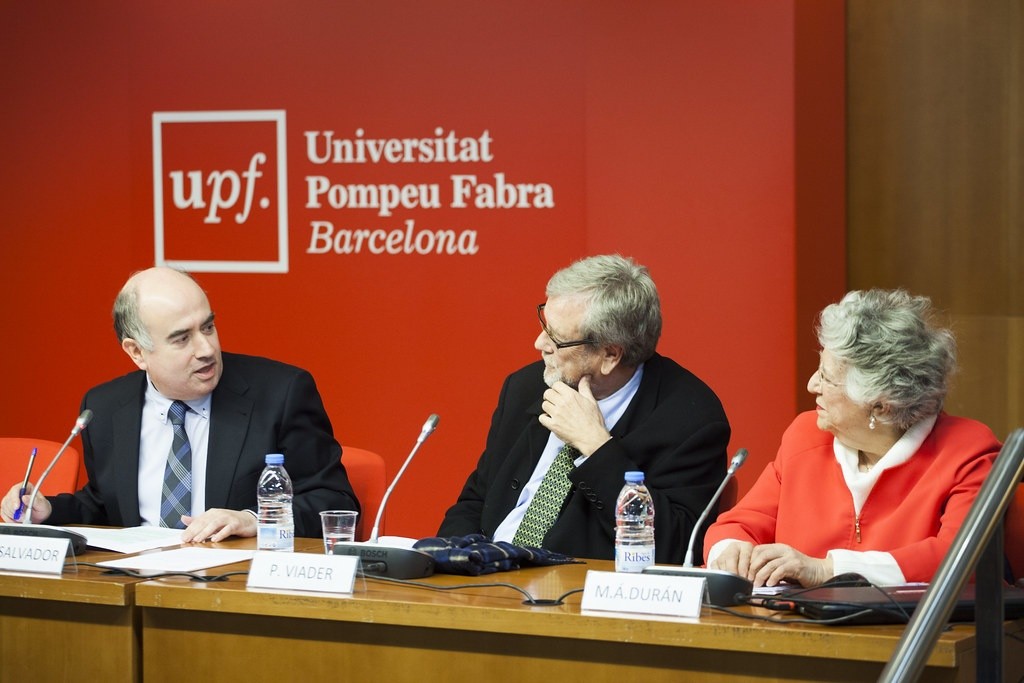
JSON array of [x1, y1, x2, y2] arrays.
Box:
[[702, 290, 1024, 601], [435, 255, 731, 566], [0, 267, 362, 543]]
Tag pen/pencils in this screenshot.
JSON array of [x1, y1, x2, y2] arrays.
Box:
[[12, 447, 38, 520]]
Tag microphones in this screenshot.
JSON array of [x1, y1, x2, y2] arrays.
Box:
[[333, 413, 439, 579], [643, 448, 753, 607], [0, 409, 93, 558]]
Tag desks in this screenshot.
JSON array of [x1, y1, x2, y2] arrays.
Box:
[[135, 545, 1024, 683], [0, 525, 323, 683]]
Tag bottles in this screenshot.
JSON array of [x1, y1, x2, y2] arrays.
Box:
[[257, 453, 294, 553], [615, 471, 656, 574]]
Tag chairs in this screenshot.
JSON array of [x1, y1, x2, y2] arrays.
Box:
[[341, 446, 386, 542], [0, 437, 79, 523]]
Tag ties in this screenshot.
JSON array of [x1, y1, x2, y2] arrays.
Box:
[[159, 401, 192, 529], [512, 444, 583, 549]]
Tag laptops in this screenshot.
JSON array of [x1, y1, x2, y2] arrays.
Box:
[[781, 582, 1024, 626]]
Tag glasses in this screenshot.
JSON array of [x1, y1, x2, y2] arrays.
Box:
[[537, 304, 600, 356]]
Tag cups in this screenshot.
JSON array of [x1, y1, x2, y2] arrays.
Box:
[[319, 510, 358, 555]]
